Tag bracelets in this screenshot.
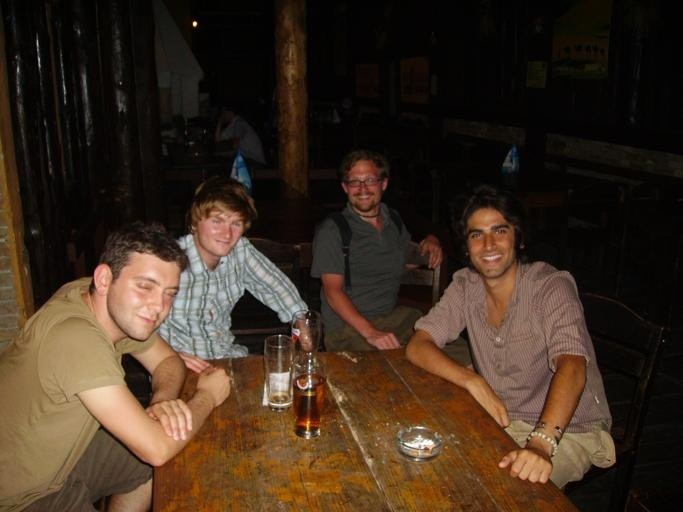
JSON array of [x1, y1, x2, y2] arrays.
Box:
[[526, 432, 557, 456], [534, 422, 564, 436]]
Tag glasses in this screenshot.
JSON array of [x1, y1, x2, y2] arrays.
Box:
[[344, 178, 383, 187]]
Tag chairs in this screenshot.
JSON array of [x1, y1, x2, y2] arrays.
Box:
[[302, 243, 440, 315], [228, 239, 307, 336], [563, 293, 669, 511]]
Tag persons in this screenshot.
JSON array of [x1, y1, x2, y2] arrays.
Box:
[[155, 176, 313, 374], [0, 222, 230, 512], [405, 187, 616, 489], [215, 107, 264, 161], [311, 151, 473, 367]]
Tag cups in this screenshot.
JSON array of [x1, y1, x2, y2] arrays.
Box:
[[292, 354, 328, 440], [263, 335, 295, 413], [290, 309, 322, 375]]
